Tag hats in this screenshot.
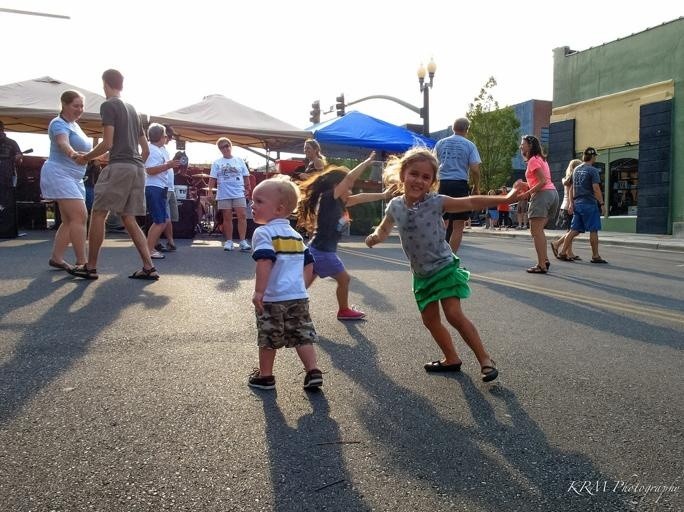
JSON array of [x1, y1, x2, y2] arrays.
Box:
[[163, 124, 181, 136], [585, 147, 600, 156]]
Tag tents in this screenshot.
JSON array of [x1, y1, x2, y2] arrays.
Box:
[[0, 75, 149, 140], [305, 109, 437, 161], [148, 92, 316, 178]]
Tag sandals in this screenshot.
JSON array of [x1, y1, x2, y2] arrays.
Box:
[[71, 264, 99, 280], [151, 253, 165, 259], [546, 258, 550, 269], [551, 242, 558, 258], [527, 265, 548, 273], [571, 256, 581, 260], [557, 253, 574, 261], [128, 267, 160, 279], [591, 256, 608, 263]]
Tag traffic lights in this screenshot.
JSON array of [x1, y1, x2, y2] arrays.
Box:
[[334, 92, 346, 118], [309, 99, 322, 125], [337, 94, 345, 118], [311, 100, 320, 125]]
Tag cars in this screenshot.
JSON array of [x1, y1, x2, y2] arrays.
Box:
[[493, 203, 545, 229], [474, 213, 492, 228]]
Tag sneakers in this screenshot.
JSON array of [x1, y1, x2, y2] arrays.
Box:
[[425, 360, 462, 373], [49, 258, 69, 269], [248, 368, 277, 390], [521, 226, 527, 230], [224, 240, 234, 251], [516, 225, 522, 229], [482, 359, 500, 381], [304, 369, 324, 389], [240, 240, 252, 250], [156, 244, 167, 252], [337, 307, 366, 320], [168, 241, 176, 251]]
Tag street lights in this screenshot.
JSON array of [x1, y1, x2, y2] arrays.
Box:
[[416, 57, 436, 140], [418, 58, 436, 139]]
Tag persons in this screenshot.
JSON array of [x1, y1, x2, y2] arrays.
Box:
[[247, 178, 324, 391], [298, 138, 325, 180], [39, 90, 108, 271], [433, 118, 482, 255], [299, 150, 398, 320], [1, 121, 25, 239], [497, 186, 510, 230], [154, 124, 182, 252], [550, 159, 583, 261], [516, 135, 560, 275], [145, 123, 182, 260], [207, 137, 252, 251], [556, 147, 608, 264], [515, 182, 529, 230], [486, 188, 499, 230], [66, 69, 161, 281], [366, 148, 527, 383]]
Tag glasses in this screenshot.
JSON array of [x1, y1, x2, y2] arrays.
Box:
[[220, 143, 230, 149]]
